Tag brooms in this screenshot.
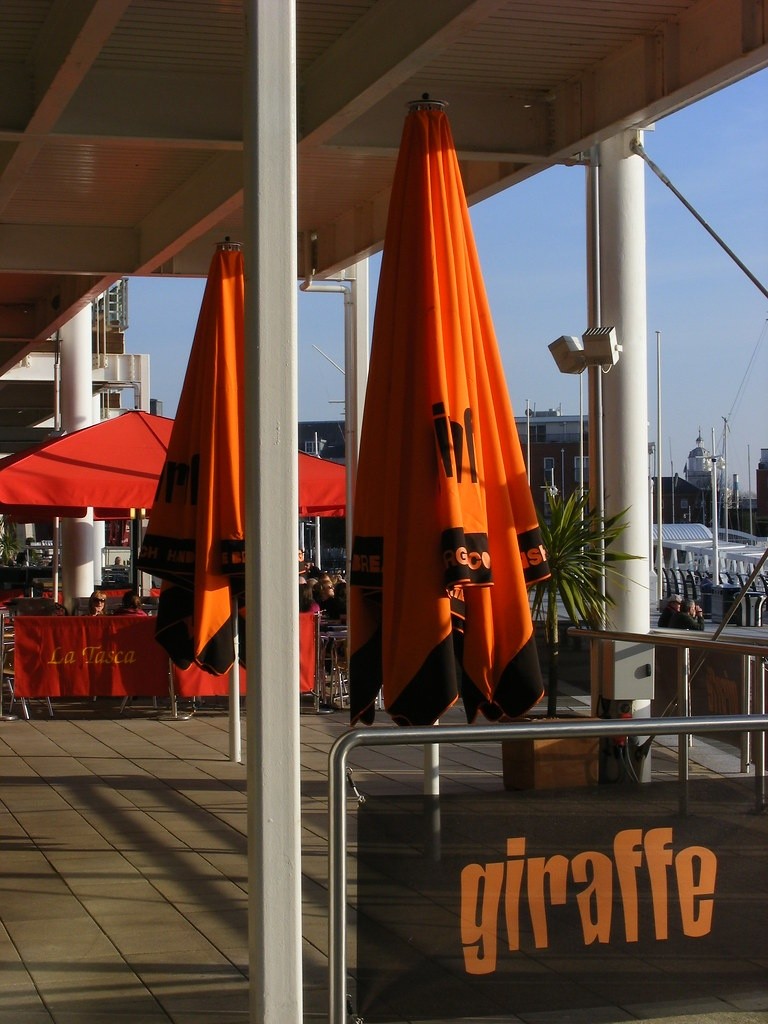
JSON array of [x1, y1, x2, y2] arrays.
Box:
[[615, 544, 768, 786]]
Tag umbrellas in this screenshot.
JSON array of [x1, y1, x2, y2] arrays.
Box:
[[350, 91, 554, 867], [138, 232, 253, 766], [0, 404, 347, 616]]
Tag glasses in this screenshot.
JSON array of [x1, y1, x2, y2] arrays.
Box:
[[93, 597, 106, 602]]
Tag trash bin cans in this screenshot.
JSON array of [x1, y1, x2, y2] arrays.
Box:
[[700, 583, 766, 627]]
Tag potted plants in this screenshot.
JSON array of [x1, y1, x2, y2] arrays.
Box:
[[502, 475, 653, 795]]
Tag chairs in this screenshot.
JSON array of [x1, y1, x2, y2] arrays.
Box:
[[1, 597, 68, 722], [320, 625, 349, 710]]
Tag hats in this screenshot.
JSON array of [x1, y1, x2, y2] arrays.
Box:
[[667, 594, 683, 603]]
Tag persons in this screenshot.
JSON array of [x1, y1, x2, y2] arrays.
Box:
[[81, 590, 107, 616], [669, 599, 705, 631], [16, 538, 36, 565], [112, 555, 125, 572], [657, 594, 682, 628], [112, 590, 148, 615], [298, 548, 347, 677]]
